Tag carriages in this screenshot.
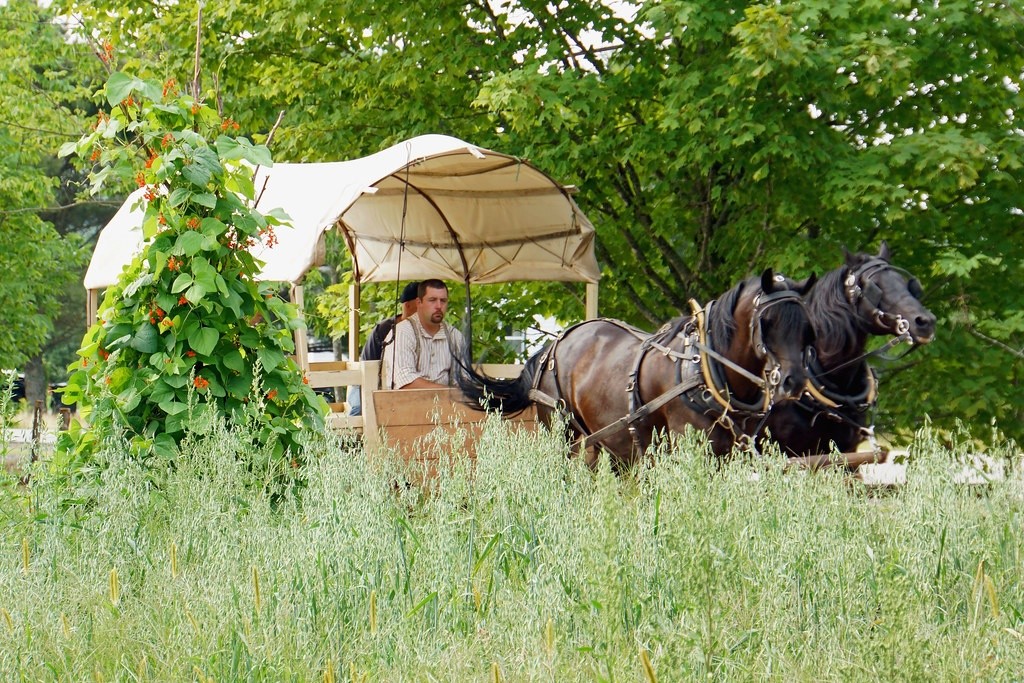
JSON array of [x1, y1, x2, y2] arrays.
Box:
[[83, 132, 936, 488]]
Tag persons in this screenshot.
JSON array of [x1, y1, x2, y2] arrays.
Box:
[[349, 282, 420, 415], [380, 278, 469, 389]]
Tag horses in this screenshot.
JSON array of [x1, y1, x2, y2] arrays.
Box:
[[450, 241, 937, 477]]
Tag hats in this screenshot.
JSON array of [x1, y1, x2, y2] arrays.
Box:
[[400, 282, 420, 302]]
